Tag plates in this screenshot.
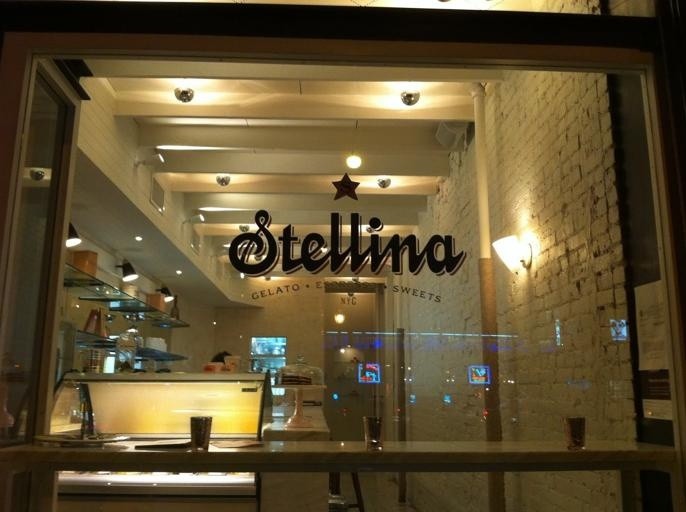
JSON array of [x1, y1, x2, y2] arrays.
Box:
[[33, 435, 130, 444]]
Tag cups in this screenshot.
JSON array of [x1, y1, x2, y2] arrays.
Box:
[[191, 417, 212, 453], [362, 416, 384, 456], [563, 416, 587, 450]]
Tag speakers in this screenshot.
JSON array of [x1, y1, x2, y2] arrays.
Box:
[[435, 121, 470, 150]]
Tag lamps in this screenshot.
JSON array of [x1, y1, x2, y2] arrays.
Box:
[[217, 175, 231, 186], [378, 178, 391, 188], [115, 262, 137, 282], [401, 89, 420, 106], [66, 223, 81, 247], [240, 223, 249, 232], [156, 286, 174, 303], [174, 87, 194, 103], [492, 237, 534, 275]]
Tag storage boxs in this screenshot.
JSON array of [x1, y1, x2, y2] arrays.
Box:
[[149, 292, 167, 311], [75, 250, 98, 273]]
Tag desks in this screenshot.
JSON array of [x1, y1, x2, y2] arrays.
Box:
[[0, 441, 685, 511]]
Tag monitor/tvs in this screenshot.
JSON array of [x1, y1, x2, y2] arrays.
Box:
[[358, 362, 380, 384], [249, 335, 287, 356], [468, 365, 491, 386], [250, 357, 287, 386]]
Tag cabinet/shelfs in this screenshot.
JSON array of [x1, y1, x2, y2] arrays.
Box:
[[61, 263, 190, 363]]
